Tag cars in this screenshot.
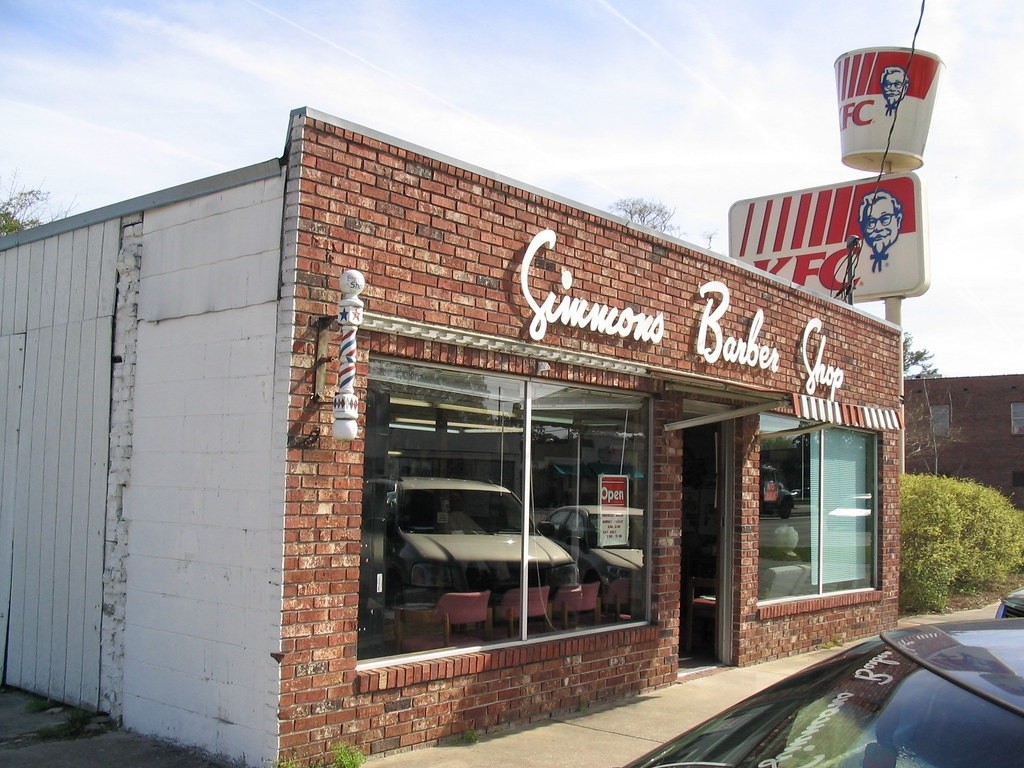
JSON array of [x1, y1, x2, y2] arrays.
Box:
[[619, 616, 1024, 768], [535, 504, 645, 614], [791, 486, 809, 500]]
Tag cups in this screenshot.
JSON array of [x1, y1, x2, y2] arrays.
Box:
[[833, 47, 948, 173]]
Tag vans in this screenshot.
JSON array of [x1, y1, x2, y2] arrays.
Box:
[[759, 465, 795, 520]]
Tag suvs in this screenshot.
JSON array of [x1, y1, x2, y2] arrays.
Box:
[[360, 474, 581, 630]]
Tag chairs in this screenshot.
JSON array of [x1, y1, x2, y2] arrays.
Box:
[[603, 577, 630, 624], [548, 581, 603, 632], [393, 589, 494, 655], [493, 585, 552, 643], [684, 576, 720, 655], [757, 563, 838, 600]]
